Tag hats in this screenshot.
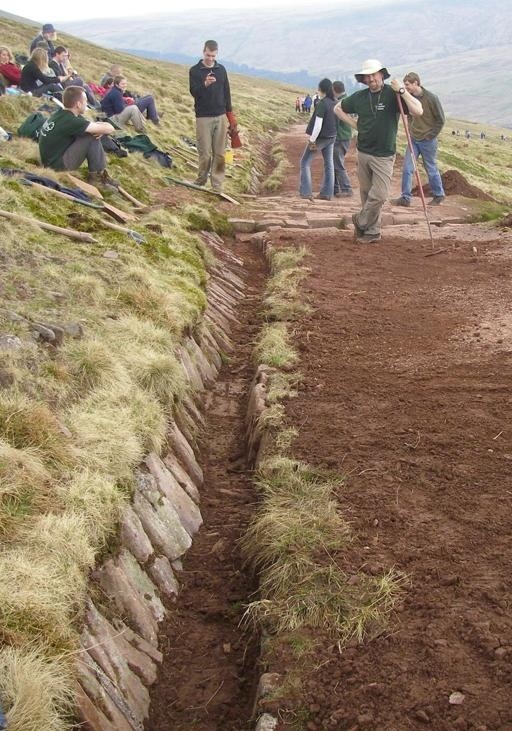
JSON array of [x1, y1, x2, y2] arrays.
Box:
[[353, 59, 391, 85], [41, 23, 56, 34]]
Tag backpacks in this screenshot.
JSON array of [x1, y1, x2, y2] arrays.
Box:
[[17, 111, 48, 139]]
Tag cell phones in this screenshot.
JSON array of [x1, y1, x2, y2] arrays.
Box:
[[209, 73, 215, 77]]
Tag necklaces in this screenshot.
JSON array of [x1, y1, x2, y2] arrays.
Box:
[[369, 90, 382, 119]]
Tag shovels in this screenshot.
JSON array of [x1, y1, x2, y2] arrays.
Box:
[[163, 175, 242, 205], [16, 178, 127, 224]]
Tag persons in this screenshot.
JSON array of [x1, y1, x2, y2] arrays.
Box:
[[299, 79, 337, 201], [295, 92, 321, 113], [188, 40, 237, 193], [333, 60, 424, 244], [0, 24, 162, 133], [38, 86, 120, 194], [452, 130, 507, 141], [332, 81, 354, 198], [389, 72, 446, 206]]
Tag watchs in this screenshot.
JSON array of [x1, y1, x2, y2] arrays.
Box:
[[399, 87, 405, 94]]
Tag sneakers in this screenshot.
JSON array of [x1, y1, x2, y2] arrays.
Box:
[[335, 191, 353, 198], [193, 178, 207, 186], [87, 171, 118, 195], [298, 194, 313, 200], [352, 213, 364, 240], [426, 194, 447, 206], [314, 194, 332, 200], [389, 197, 411, 207], [211, 186, 223, 195], [356, 233, 382, 245], [98, 168, 120, 188]]
